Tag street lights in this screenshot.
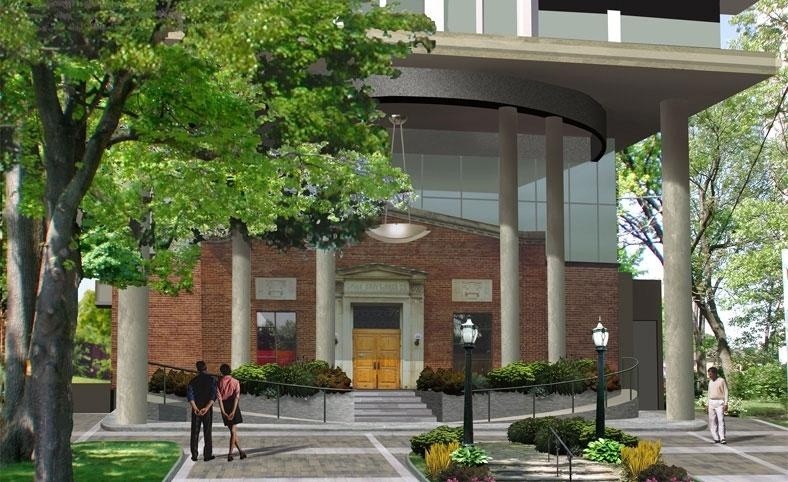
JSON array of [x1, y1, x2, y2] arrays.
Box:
[[590, 316, 609, 443], [460, 315, 479, 449]]
